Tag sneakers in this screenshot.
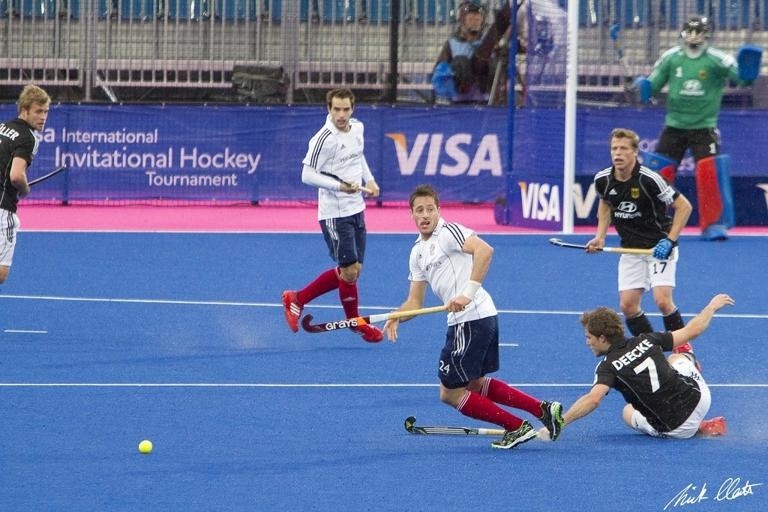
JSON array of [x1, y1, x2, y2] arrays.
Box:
[[282, 290, 304, 332], [346, 317, 383, 342], [672, 342, 701, 372], [537, 399, 564, 441], [702, 224, 728, 241], [698, 416, 726, 436], [491, 420, 537, 449]]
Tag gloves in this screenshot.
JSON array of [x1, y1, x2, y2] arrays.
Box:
[[652, 238, 674, 260]]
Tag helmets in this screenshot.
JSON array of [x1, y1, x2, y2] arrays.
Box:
[[680, 15, 714, 49], [456, 2, 486, 33]]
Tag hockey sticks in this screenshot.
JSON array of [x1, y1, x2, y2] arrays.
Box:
[[320, 170, 374, 195], [549, 238, 652, 255], [405, 416, 539, 436], [302, 305, 448, 333]]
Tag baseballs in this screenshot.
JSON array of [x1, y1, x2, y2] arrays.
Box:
[[139, 440, 152, 454]]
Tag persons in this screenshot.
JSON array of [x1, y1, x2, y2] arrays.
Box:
[[383, 185, 564, 449], [635, 13, 762, 240], [0, 86, 51, 284], [282, 89, 383, 343], [585, 129, 692, 334], [434, 2, 497, 104], [537, 292, 734, 440]]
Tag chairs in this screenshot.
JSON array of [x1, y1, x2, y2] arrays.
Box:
[[1, 0, 767, 35]]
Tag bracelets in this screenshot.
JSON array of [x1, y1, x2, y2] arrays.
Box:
[[461, 280, 481, 299]]
